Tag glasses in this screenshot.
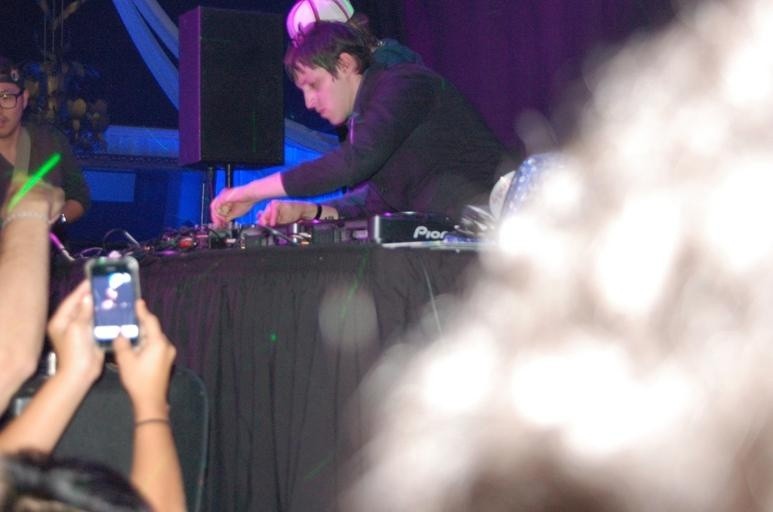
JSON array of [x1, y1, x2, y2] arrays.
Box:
[[0, 89, 26, 109]]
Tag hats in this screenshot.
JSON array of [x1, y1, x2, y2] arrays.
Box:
[[0, 56, 27, 91]]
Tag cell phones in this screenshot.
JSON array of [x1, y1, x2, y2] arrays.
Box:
[[85, 255, 142, 353]]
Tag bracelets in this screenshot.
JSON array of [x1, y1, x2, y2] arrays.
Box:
[[59, 211, 67, 224], [134, 417, 171, 427], [313, 202, 322, 219], [1, 209, 49, 225]]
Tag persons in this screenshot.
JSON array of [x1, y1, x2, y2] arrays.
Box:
[[209, 18, 504, 232], [0, 59, 91, 224], [0, 276, 188, 512], [0, 152, 66, 417]]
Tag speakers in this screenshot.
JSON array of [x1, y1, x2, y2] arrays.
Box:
[[177, 4, 286, 170]]
